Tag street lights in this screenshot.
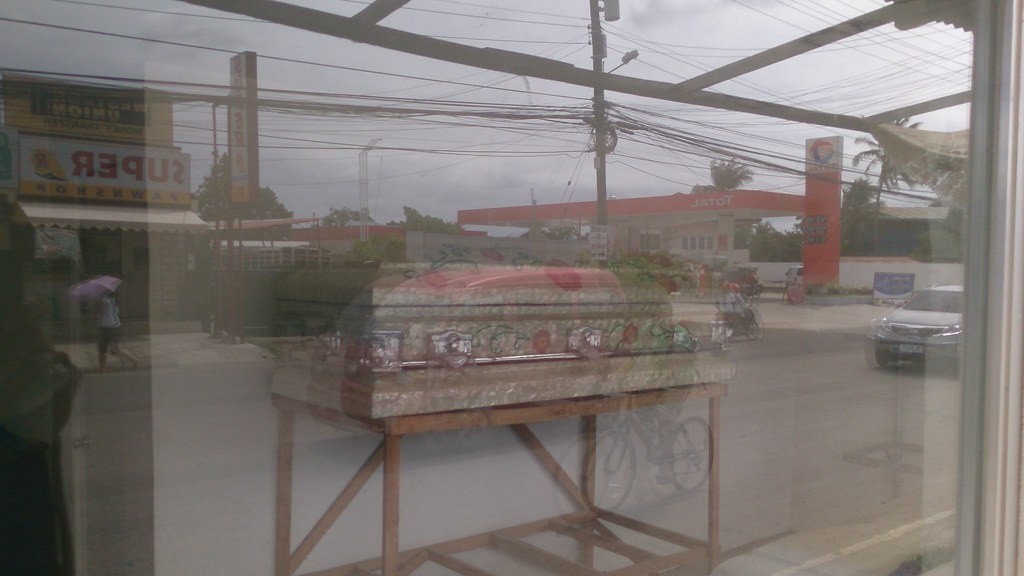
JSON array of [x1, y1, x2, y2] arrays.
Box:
[[596, 47, 640, 269]]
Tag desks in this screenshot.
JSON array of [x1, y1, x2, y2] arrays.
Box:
[[263, 381, 728, 576]]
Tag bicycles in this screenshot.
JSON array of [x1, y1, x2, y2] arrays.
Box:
[[708, 294, 766, 351], [723, 263, 764, 299], [577, 387, 713, 517]]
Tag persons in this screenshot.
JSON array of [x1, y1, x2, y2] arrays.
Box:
[[716, 279, 755, 339], [605, 299, 697, 485], [89, 285, 141, 374], [0, 201, 76, 576]]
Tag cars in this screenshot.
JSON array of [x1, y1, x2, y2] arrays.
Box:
[[784, 266, 804, 284], [875, 286, 964, 373]]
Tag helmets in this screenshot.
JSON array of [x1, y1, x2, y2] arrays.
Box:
[[721, 281, 730, 292], [729, 284, 740, 293]]
